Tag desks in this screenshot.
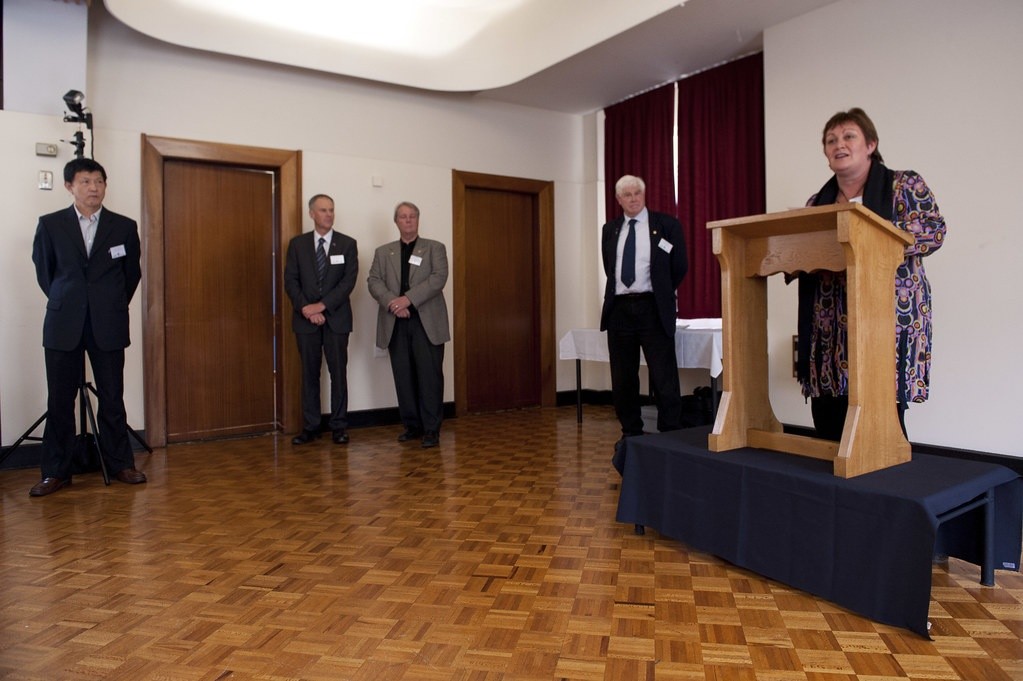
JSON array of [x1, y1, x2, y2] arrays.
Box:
[[559, 328, 723, 424], [615, 426, 1023, 642]]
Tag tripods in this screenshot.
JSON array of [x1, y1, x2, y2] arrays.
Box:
[[1, 131, 154, 488]]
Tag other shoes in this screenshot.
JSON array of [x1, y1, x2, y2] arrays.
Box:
[[614, 433, 644, 451]]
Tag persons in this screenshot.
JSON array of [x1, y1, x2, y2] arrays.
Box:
[[367, 202, 451, 448], [598, 175, 689, 438], [283, 194, 361, 446], [785, 107, 947, 444], [29, 158, 149, 498]]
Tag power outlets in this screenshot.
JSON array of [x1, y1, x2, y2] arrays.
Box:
[[35, 143, 58, 158], [374, 343, 388, 358]]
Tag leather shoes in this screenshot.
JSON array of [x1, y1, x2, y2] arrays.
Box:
[[332, 428, 349, 443], [398, 428, 424, 441], [112, 466, 146, 483], [291, 428, 322, 444], [422, 429, 439, 448], [29, 476, 72, 496]]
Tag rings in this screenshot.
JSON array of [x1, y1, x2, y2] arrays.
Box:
[[396, 305, 399, 308]]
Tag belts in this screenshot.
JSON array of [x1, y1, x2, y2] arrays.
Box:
[[613, 292, 655, 298]]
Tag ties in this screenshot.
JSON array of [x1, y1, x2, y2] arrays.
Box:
[[316, 237, 326, 299], [621, 219, 637, 288]]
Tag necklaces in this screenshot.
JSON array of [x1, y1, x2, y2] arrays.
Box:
[[839, 185, 864, 203]]
